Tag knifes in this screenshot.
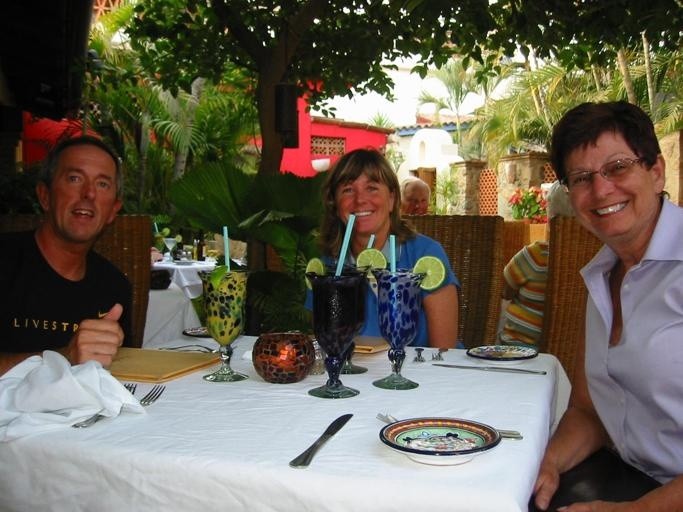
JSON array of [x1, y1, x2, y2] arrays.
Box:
[[432, 364, 547, 375], [289, 414, 354, 469]]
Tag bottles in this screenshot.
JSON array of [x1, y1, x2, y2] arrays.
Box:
[[198, 229, 206, 261]]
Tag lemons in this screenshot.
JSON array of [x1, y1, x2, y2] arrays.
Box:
[[304, 257, 324, 290], [174, 235, 182, 242], [357, 248, 388, 279], [210, 266, 227, 289], [163, 228, 171, 237], [413, 256, 446, 290]]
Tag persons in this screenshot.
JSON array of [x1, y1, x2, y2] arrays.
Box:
[[495, 180, 577, 350], [529, 100, 683, 512], [0, 136, 133, 378], [150, 247, 163, 262], [399, 176, 431, 215], [304, 148, 461, 349]]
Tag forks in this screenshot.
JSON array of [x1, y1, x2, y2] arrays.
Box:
[[159, 344, 237, 353], [72, 384, 166, 428]]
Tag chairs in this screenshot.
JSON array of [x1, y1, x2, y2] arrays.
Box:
[[542, 215, 610, 411], [399, 216, 509, 350], [89, 215, 154, 348]]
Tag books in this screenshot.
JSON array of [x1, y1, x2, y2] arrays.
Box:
[[106, 348, 223, 384], [353, 336, 391, 353]]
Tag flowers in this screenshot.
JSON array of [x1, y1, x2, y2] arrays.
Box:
[[508, 184, 548, 226]]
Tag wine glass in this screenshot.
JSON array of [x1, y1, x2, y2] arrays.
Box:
[[198, 270, 252, 382], [305, 272, 364, 399], [371, 268, 426, 390], [163, 238, 178, 263]]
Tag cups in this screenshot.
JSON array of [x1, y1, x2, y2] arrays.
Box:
[[152, 232, 165, 255]]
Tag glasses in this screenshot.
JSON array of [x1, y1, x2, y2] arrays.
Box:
[[558, 156, 645, 190]]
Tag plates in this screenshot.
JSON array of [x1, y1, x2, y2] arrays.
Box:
[[379, 417, 502, 466], [467, 344, 539, 365], [182, 326, 217, 342]]
